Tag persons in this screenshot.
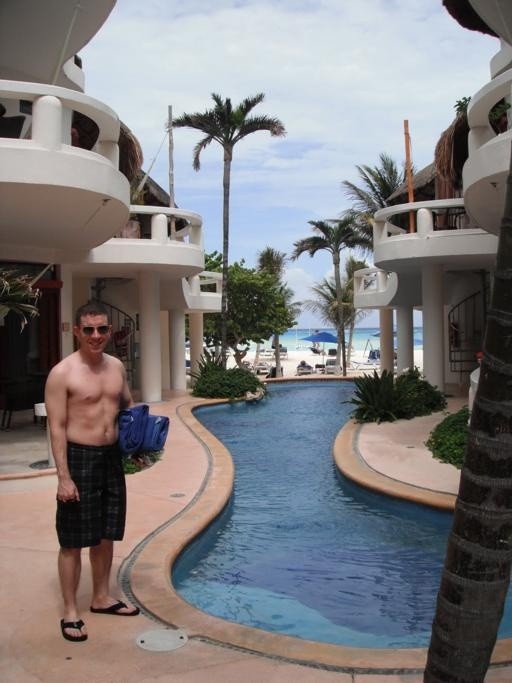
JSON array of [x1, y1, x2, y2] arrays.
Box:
[[313, 330, 320, 349], [271, 335, 282, 348], [298, 360, 311, 369], [45, 302, 140, 642]]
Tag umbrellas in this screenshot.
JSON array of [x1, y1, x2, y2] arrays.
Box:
[[299, 332, 347, 364]]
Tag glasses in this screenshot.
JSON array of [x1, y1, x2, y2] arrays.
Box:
[[82, 324, 110, 334]]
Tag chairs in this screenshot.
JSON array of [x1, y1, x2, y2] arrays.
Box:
[[243, 347, 342, 376]]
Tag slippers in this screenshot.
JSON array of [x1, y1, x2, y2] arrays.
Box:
[[61, 617, 88, 641], [87, 598, 140, 616]]
